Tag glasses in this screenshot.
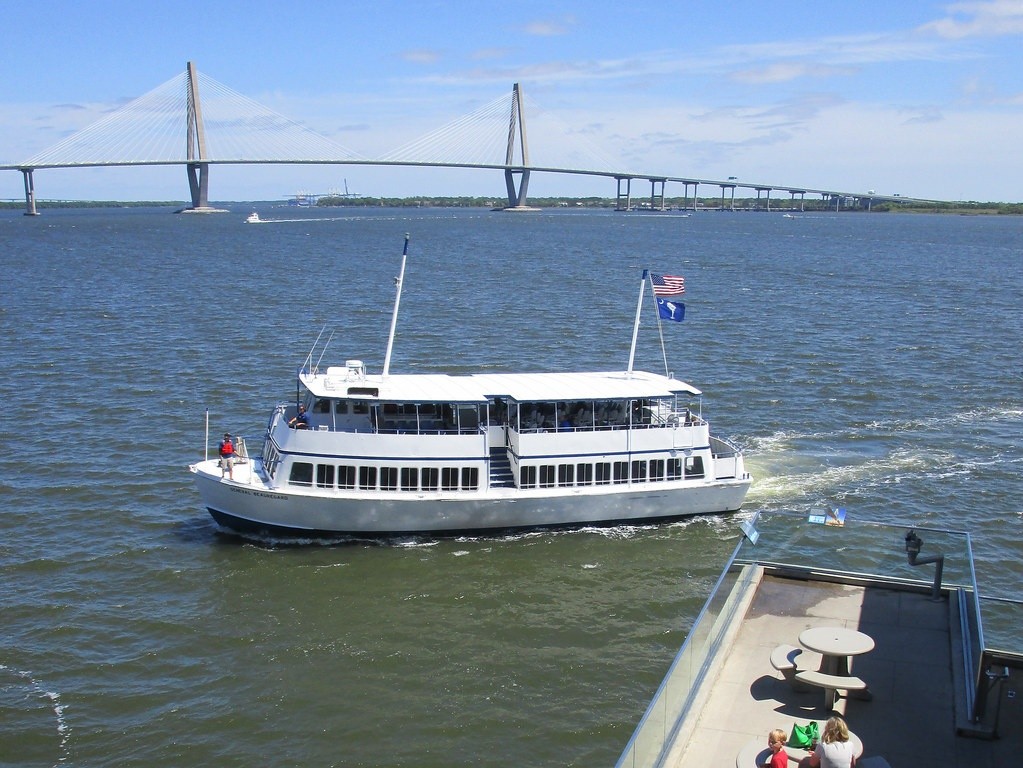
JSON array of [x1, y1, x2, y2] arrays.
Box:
[[768, 739, 781, 745]]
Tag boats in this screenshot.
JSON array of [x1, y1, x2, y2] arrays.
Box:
[[186, 229, 755, 538]]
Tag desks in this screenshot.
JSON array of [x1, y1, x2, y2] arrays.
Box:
[[789, 720, 863, 761], [799, 626, 875, 700]]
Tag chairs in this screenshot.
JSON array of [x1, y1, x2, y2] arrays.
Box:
[[384, 420, 443, 429], [521, 408, 628, 433]]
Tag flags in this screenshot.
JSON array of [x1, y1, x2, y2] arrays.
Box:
[[657, 297, 687, 323], [650, 274, 685, 295]]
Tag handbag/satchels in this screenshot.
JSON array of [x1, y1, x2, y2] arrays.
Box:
[[788, 722, 820, 747]]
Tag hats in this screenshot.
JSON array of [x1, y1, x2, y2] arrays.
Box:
[[224, 433, 232, 437]]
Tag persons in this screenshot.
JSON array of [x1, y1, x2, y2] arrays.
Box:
[[809, 716, 856, 768], [219, 433, 240, 481], [760, 729, 788, 768], [559, 415, 572, 432], [290, 406, 308, 428]]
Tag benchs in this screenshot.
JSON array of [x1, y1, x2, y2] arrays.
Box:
[[795, 670, 867, 709], [770, 644, 807, 692], [735, 740, 773, 767]]
